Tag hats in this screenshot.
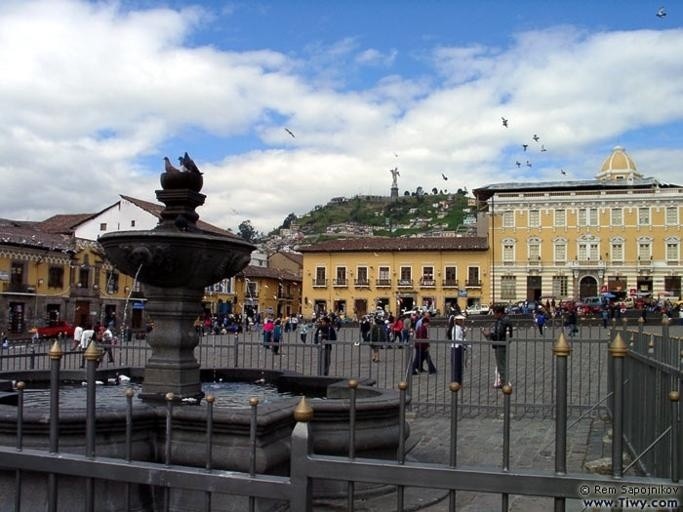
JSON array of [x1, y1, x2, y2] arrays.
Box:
[[453, 314, 466, 320]]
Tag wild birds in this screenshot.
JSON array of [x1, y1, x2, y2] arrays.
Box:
[[516, 134, 547, 168], [441, 174, 447, 181], [656, 6, 666, 19], [501, 117, 508, 128], [284, 128, 295, 137], [561, 170, 565, 174]]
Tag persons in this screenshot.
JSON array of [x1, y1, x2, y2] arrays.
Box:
[[0, 289, 683, 390], [390, 167, 401, 188]]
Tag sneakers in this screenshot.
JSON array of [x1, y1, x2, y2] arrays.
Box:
[[412, 367, 437, 375]]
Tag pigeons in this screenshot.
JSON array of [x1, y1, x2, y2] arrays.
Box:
[[162, 152, 199, 173]]
